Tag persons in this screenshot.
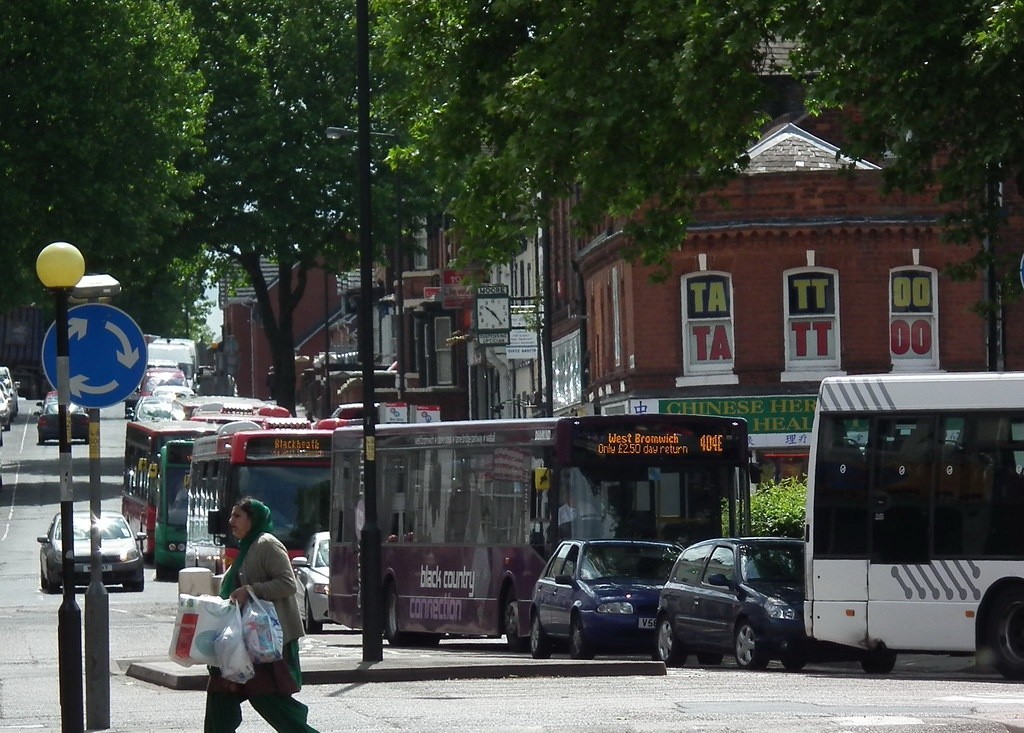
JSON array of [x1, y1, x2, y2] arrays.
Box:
[[205, 497, 319, 732]]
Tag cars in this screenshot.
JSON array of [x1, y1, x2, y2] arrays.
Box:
[[36, 512, 147, 594], [290, 527, 337, 633], [526, 537, 723, 665], [1, 320, 288, 490], [648, 535, 898, 675]]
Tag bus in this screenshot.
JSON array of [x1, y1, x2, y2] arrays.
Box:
[[799, 372, 1024, 680], [185, 413, 355, 595], [329, 412, 761, 644], [122, 402, 318, 578]]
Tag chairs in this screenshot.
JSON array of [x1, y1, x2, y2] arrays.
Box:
[[829, 433, 990, 501]]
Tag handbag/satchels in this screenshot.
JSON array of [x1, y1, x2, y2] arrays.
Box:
[[241, 584, 284, 665], [212, 598, 255, 685]]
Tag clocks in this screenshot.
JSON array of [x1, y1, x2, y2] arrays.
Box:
[[476, 293, 512, 335]]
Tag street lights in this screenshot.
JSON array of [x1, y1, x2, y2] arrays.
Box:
[[33, 237, 87, 728]]
